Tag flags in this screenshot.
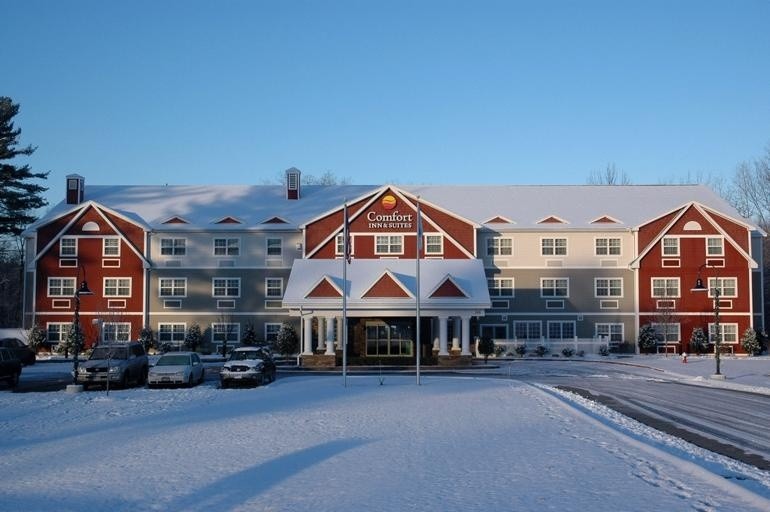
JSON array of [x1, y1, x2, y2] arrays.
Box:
[[346, 212, 352, 264], [419, 209, 424, 251]]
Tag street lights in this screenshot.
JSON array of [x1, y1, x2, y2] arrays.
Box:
[[75, 265, 96, 385], [694, 264, 720, 374]]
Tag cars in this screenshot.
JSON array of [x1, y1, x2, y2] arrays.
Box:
[[0, 338, 35, 385], [219, 346, 275, 387], [148, 351, 204, 388], [77, 341, 148, 389]]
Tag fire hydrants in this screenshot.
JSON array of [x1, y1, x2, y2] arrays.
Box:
[[681, 352, 687, 363]]
